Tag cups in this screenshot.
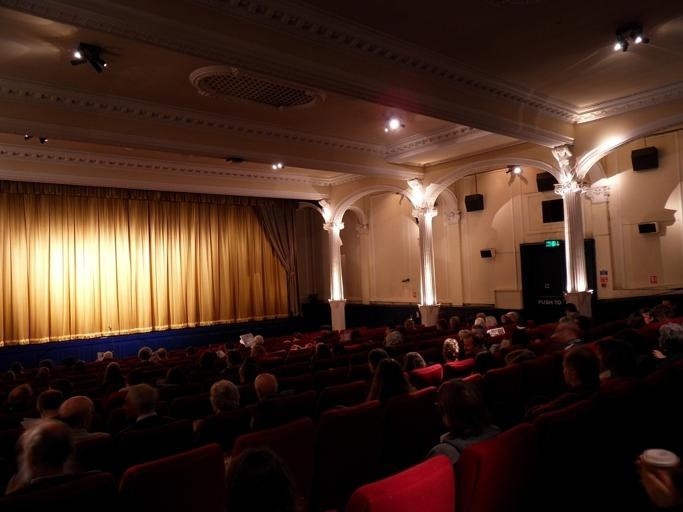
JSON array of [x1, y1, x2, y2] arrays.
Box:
[[643, 447, 678, 479]]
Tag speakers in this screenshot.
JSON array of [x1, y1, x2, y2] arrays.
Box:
[[465, 195, 483, 212], [480, 250, 496, 258], [632, 147, 658, 171], [638, 223, 657, 234]]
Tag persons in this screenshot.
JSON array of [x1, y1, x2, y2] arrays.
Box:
[[2, 298, 683, 511]]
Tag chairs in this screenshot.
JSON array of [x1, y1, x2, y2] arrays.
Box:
[[0, 311, 683, 511]]
